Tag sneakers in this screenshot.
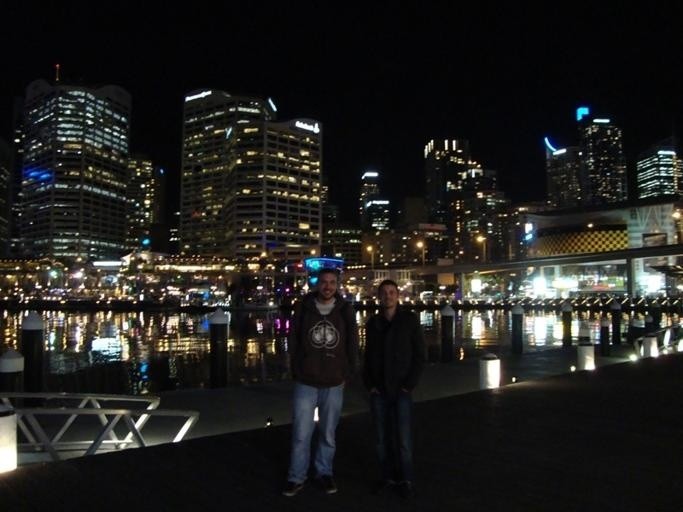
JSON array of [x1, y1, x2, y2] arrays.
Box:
[[371, 476, 400, 496], [319, 475, 337, 495], [398, 480, 415, 500], [282, 481, 304, 497]]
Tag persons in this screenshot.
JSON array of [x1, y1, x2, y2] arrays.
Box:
[[359, 279, 423, 500], [280, 268, 357, 497]]
